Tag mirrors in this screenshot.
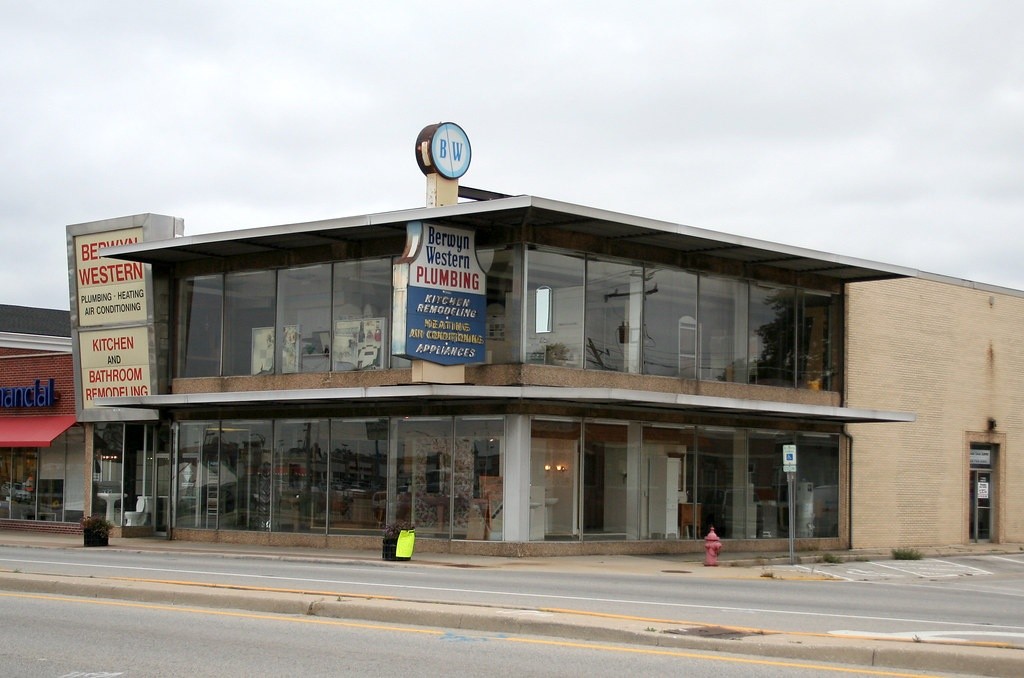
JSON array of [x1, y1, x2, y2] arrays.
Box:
[[535, 285, 553, 334]]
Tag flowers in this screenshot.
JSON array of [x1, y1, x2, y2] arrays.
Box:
[[384, 520, 412, 538], [79, 512, 114, 539]]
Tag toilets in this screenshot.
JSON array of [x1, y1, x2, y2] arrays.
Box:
[[123, 496, 152, 527]]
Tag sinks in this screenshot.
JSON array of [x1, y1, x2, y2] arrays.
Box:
[[97, 492, 127, 502]]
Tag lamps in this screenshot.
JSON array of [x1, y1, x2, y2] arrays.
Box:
[[545, 464, 554, 471], [556, 465, 566, 471]]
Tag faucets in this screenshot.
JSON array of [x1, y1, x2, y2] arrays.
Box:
[[106, 488, 111, 493]]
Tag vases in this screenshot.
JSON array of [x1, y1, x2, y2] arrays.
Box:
[[83, 527, 109, 547], [382, 537, 411, 561]]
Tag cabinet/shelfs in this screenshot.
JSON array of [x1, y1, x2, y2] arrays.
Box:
[[678, 503, 702, 539], [648, 455, 678, 540], [206, 461, 239, 529]]
